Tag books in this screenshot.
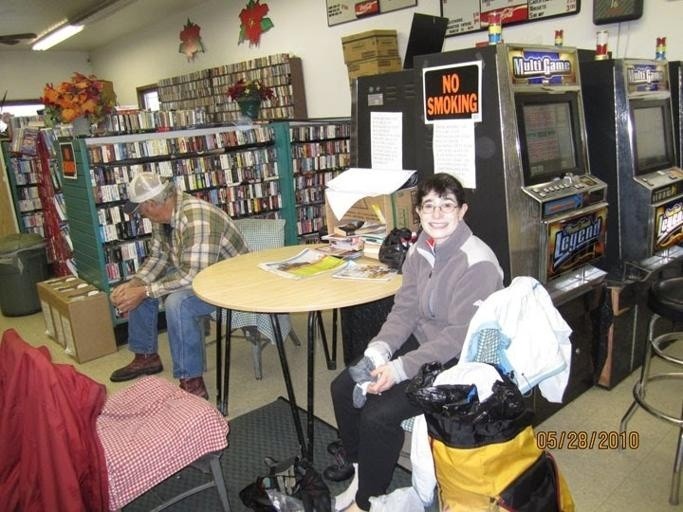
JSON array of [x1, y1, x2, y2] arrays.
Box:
[[0, 110, 77, 276], [106, 111, 207, 133], [287, 116, 357, 245], [316, 220, 391, 266], [148, 51, 294, 120], [101, 223, 153, 283], [86, 127, 282, 222]]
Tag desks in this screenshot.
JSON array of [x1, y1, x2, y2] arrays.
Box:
[[192, 243, 406, 463]]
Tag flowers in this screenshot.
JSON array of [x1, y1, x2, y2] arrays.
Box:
[[41, 70, 117, 125], [224, 78, 276, 101]]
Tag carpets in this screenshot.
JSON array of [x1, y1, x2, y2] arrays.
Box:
[[111, 397, 412, 511]]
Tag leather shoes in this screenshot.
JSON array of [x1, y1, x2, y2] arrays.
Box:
[[328, 439, 344, 455], [111, 354, 162, 382], [180, 377, 209, 400], [323, 463, 355, 482]]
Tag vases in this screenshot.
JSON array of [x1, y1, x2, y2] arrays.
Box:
[[237, 96, 260, 120], [71, 115, 91, 138]]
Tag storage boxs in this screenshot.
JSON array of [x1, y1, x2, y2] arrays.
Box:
[[340, 28, 399, 64], [36, 274, 118, 364], [326, 182, 420, 236], [346, 57, 402, 84]]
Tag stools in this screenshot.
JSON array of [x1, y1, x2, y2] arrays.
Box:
[[617, 277, 682, 506]]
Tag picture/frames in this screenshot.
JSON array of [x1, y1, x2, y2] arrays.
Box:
[[592, 0, 643, 24], [440, 1, 481, 38], [528, 0, 580, 21], [479, 0, 528, 28]]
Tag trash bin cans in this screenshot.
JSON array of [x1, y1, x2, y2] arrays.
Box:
[[1, 231, 48, 318]]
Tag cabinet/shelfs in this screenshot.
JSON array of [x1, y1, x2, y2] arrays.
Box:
[[157, 54, 308, 119], [0, 121, 57, 241], [56, 121, 290, 348], [285, 115, 357, 246], [34, 122, 242, 277]]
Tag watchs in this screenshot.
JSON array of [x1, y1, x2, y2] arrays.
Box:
[[145, 286, 152, 298]]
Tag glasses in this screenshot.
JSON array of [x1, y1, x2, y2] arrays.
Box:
[[418, 202, 461, 213]]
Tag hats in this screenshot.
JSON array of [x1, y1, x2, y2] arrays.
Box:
[[123, 173, 170, 216]]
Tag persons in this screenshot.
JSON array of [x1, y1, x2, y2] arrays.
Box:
[[110, 173, 253, 402], [331, 173, 505, 512]]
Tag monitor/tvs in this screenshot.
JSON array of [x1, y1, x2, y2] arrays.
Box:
[[514, 92, 586, 186], [629, 98, 676, 177]]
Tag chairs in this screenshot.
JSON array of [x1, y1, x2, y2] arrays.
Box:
[[398, 277, 541, 474], [1, 331, 230, 511], [205, 219, 301, 380]]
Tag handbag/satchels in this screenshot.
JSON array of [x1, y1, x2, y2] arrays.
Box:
[[238, 457, 331, 512]]
